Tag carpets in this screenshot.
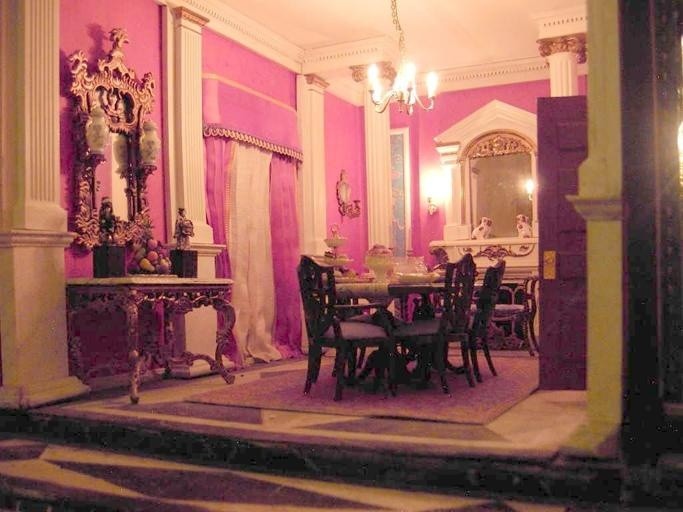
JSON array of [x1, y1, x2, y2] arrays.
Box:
[[184, 352, 539, 425]]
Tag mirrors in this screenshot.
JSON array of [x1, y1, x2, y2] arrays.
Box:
[[433, 101, 537, 239], [66, 23, 161, 246]]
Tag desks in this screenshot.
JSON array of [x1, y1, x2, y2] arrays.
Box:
[[64, 277, 236, 407]]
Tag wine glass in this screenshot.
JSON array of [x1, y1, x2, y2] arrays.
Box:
[[366, 256, 395, 283]]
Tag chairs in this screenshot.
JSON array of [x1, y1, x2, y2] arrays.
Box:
[[292, 252, 539, 400]]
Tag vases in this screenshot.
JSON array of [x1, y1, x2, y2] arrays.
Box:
[[140, 120, 159, 163], [84, 102, 110, 154]]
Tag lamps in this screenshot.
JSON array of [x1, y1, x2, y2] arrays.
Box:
[[334, 169, 362, 218], [362, 1, 437, 116]]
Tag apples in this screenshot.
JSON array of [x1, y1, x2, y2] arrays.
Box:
[[128, 239, 171, 272]]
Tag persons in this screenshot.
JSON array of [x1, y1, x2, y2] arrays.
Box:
[[173, 208, 194, 249], [98, 202, 119, 246]]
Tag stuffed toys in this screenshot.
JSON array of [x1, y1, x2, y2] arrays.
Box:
[[516, 214, 532, 239], [471, 217, 492, 239]]
